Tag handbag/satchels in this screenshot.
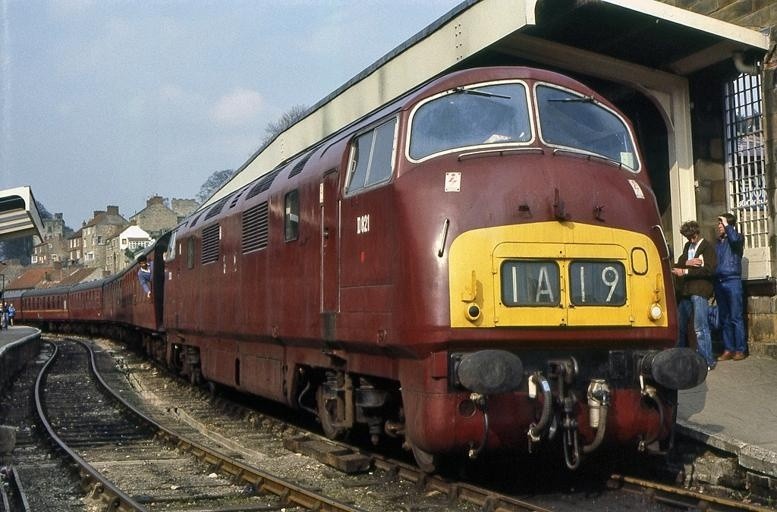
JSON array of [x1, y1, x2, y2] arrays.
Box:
[[708, 298, 719, 331]]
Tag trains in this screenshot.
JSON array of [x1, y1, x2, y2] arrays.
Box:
[[0, 57, 716, 501]]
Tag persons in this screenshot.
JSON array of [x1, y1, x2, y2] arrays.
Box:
[[713, 213, 749, 361], [0, 301, 16, 327], [670, 221, 717, 373], [136, 255, 155, 298]]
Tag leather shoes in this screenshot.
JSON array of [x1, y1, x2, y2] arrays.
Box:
[[715, 350, 732, 361], [733, 351, 747, 361]]
